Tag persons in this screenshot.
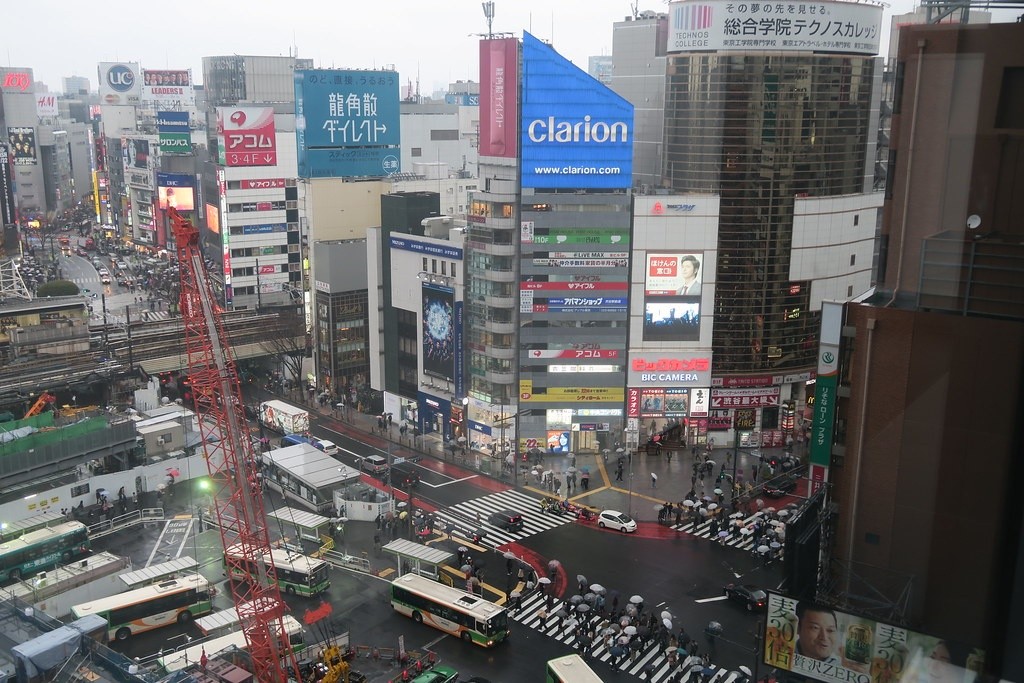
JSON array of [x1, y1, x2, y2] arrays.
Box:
[[794, 601, 871, 675], [523, 443, 632, 517], [16, 251, 54, 290], [71, 472, 174, 528], [423, 308, 454, 362], [375, 501, 453, 545], [268, 375, 337, 412], [329, 505, 344, 539], [376, 411, 408, 436], [457, 546, 748, 683], [126, 269, 175, 309], [555, 432, 569, 451], [926, 640, 977, 683], [450, 441, 466, 455], [127, 139, 140, 167], [651, 436, 798, 567], [9, 133, 34, 158], [472, 512, 481, 543], [167, 188, 183, 210], [674, 255, 701, 296]]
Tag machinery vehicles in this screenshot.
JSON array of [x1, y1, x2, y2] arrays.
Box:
[[166, 205, 366, 683]]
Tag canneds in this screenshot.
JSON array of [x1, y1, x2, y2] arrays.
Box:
[[844, 621, 873, 666]]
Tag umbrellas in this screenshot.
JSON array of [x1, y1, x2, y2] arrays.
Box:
[[531, 447, 796, 554], [739, 666, 751, 676], [630, 595, 643, 603], [385, 502, 705, 673], [662, 619, 672, 629], [661, 611, 672, 620]]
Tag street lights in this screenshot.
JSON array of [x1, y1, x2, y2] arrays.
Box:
[[625, 422, 634, 517]]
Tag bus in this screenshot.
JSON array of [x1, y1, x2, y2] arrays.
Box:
[[220, 543, 331, 598], [71, 573, 216, 642], [391, 573, 510, 650], [158, 615, 308, 683], [119, 556, 201, 593], [194, 597, 291, 643], [546, 654, 603, 683], [0, 521, 91, 586]]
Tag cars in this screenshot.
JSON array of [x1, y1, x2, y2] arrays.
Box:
[[317, 440, 339, 456], [58, 236, 142, 291], [723, 584, 767, 611], [598, 510, 638, 532], [408, 664, 459, 683]]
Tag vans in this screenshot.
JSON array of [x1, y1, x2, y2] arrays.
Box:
[[488, 511, 524, 533], [281, 435, 324, 453], [362, 455, 388, 474]]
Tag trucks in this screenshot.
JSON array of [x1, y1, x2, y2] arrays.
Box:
[[257, 399, 310, 437], [0, 614, 110, 683]]
